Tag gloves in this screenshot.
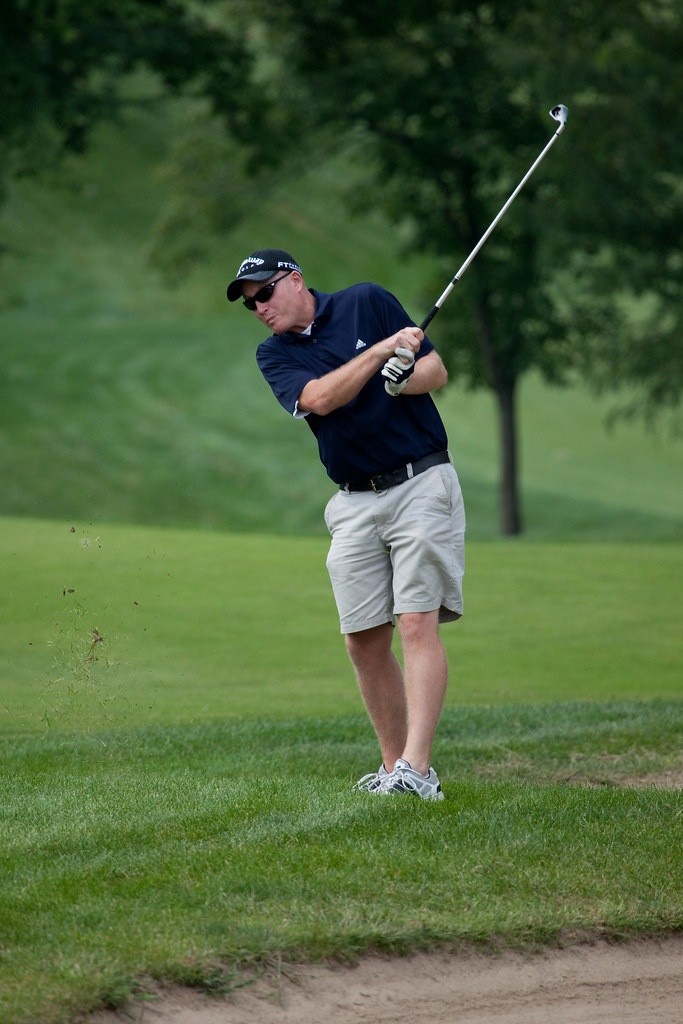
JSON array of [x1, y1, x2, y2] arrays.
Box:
[[381, 347, 416, 396]]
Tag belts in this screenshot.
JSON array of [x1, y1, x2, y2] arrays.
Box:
[[339, 450, 452, 491]]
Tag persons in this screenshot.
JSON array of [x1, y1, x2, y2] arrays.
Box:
[[227, 249, 466, 802]]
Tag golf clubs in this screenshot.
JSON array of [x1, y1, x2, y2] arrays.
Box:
[[383, 103, 569, 383]]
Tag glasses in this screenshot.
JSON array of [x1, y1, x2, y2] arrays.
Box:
[[242, 271, 292, 310]]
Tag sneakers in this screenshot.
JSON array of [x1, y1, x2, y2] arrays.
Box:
[[352, 757, 445, 801]]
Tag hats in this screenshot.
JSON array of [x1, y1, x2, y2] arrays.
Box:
[[227, 248, 302, 302]]
